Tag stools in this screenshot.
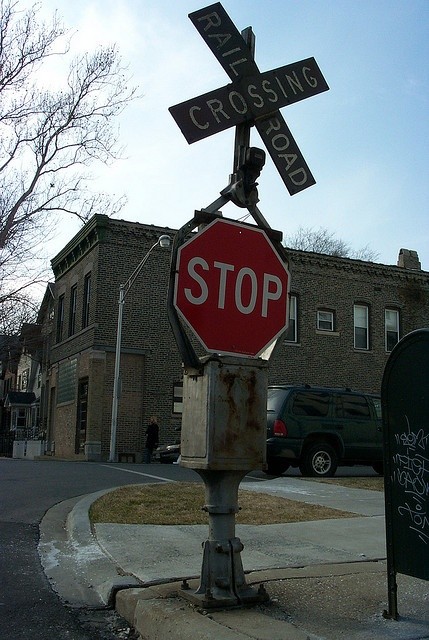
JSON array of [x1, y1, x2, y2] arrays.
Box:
[[118, 452, 135, 464]]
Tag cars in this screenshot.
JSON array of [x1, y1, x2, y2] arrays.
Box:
[[152, 444, 180, 462]]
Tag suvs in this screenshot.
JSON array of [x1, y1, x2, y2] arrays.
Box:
[[265, 385, 387, 478]]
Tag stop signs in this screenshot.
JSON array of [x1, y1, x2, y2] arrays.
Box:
[[174, 218, 291, 359]]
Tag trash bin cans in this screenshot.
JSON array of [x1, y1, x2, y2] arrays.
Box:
[[84, 441, 100, 461]]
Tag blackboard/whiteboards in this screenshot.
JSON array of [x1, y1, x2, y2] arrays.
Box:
[[382, 328, 429, 620]]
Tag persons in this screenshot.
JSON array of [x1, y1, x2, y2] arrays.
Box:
[[145, 415, 160, 462]]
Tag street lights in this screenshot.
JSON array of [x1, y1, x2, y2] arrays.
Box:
[[108, 234, 170, 463]]
